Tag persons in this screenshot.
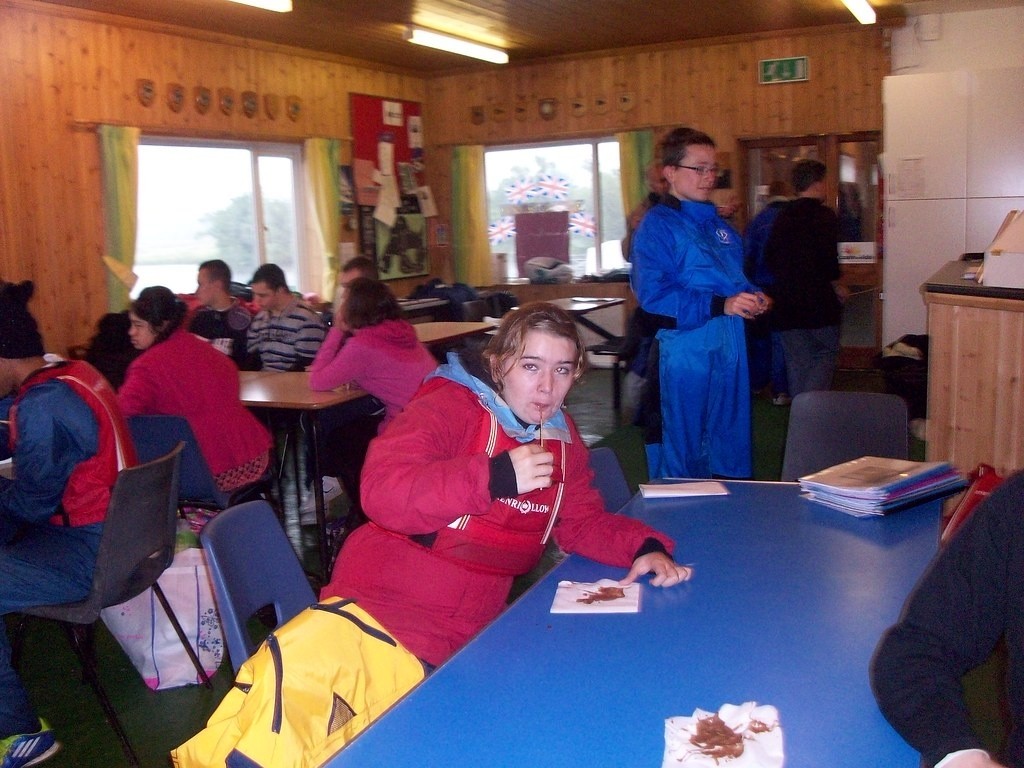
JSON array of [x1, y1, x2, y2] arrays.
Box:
[[623, 128, 856, 480], [870, 470, 1024, 768], [321, 301, 692, 678], [0, 252, 443, 768]]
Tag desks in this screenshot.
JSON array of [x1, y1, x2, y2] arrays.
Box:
[[922, 259, 1024, 480], [319, 485, 943, 768], [412, 320, 497, 348], [545, 296, 627, 342], [234, 361, 371, 569]]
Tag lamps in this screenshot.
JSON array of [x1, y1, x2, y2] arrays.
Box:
[[400, 22, 512, 65]]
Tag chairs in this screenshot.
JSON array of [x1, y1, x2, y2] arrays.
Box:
[[589, 447, 631, 515], [199, 500, 321, 676], [463, 299, 494, 321], [8, 440, 214, 767], [778, 389, 909, 482]]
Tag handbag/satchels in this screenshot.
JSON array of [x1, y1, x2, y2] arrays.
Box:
[[99, 547, 229, 691]]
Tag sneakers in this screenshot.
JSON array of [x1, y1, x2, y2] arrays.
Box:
[[0, 717, 59, 768], [300, 503, 329, 525], [298, 476, 343, 514]]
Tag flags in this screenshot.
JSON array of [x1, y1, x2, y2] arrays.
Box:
[[487, 175, 596, 244]]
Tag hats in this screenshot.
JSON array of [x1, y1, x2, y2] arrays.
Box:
[[0, 280, 45, 359]]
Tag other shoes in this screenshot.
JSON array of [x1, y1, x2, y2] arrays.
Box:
[[773, 392, 793, 405]]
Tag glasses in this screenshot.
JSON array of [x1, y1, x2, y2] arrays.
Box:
[[676, 162, 722, 176]]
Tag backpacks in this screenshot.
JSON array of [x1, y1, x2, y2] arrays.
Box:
[[67, 310, 146, 392], [228, 282, 255, 302]]
